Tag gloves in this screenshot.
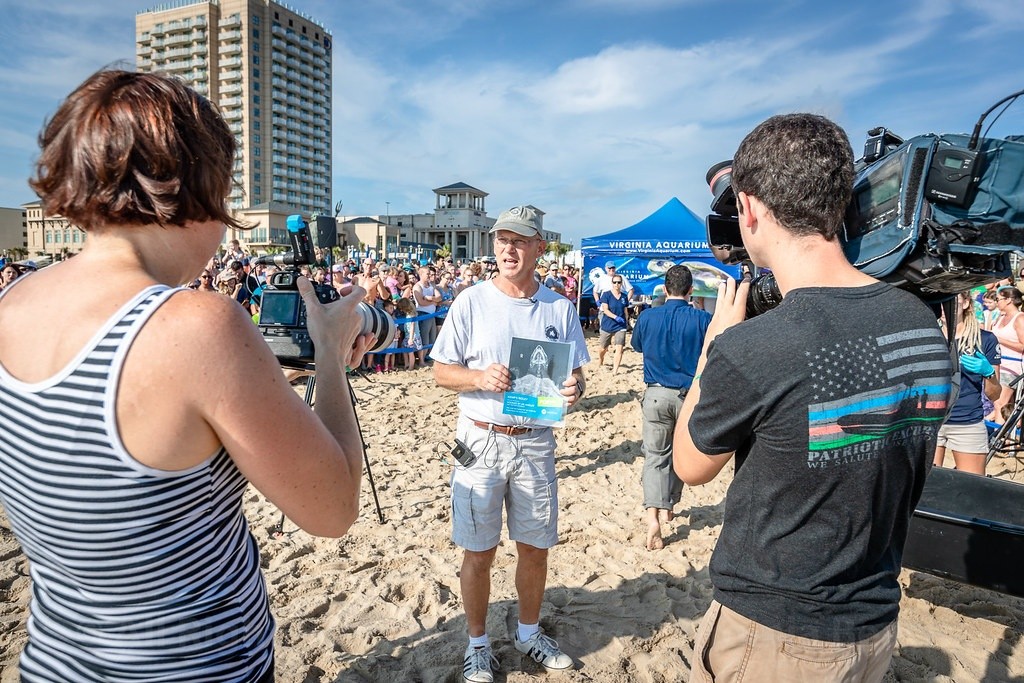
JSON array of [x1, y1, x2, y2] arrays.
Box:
[[615, 317, 625, 323], [960, 351, 995, 376]]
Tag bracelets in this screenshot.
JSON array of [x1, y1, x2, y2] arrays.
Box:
[[702, 309, 705, 311], [692, 374, 700, 382], [984, 372, 995, 379]]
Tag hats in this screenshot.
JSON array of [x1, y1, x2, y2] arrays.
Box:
[[550, 264, 558, 270], [332, 265, 343, 272], [605, 261, 616, 268], [489, 206, 544, 238], [445, 256, 453, 262], [16, 260, 38, 269]]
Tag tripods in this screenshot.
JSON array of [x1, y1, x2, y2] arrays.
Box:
[[277, 377, 385, 535]]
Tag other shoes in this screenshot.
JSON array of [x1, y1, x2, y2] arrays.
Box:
[[393, 368, 399, 373], [419, 361, 431, 368], [385, 369, 390, 374], [376, 367, 383, 374]]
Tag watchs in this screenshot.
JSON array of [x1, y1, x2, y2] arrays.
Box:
[[578, 381, 583, 397]]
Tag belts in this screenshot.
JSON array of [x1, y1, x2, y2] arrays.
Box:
[[475, 421, 532, 435], [648, 383, 662, 388]]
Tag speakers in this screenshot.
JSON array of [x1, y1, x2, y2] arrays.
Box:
[[317, 216, 337, 249]]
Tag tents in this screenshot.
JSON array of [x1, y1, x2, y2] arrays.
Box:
[[576, 198, 742, 316]]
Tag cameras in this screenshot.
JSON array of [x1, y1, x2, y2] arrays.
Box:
[[257, 270, 395, 354]]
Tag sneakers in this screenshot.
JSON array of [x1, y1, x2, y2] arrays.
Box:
[[514, 629, 574, 672], [463, 646, 499, 683]]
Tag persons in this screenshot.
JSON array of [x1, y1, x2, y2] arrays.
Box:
[[934, 291, 1002, 477], [699, 297, 716, 315], [188, 239, 593, 385], [0, 66, 377, 683], [631, 264, 713, 550], [973, 267, 1024, 448], [594, 260, 634, 352], [428, 207, 591, 683], [0, 260, 37, 292], [672, 114, 951, 683], [597, 276, 630, 373]]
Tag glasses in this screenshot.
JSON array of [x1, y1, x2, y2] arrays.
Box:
[[997, 298, 1006, 302], [202, 275, 212, 279], [613, 281, 621, 284], [494, 236, 540, 247], [447, 261, 453, 263], [17, 267, 30, 272]]
